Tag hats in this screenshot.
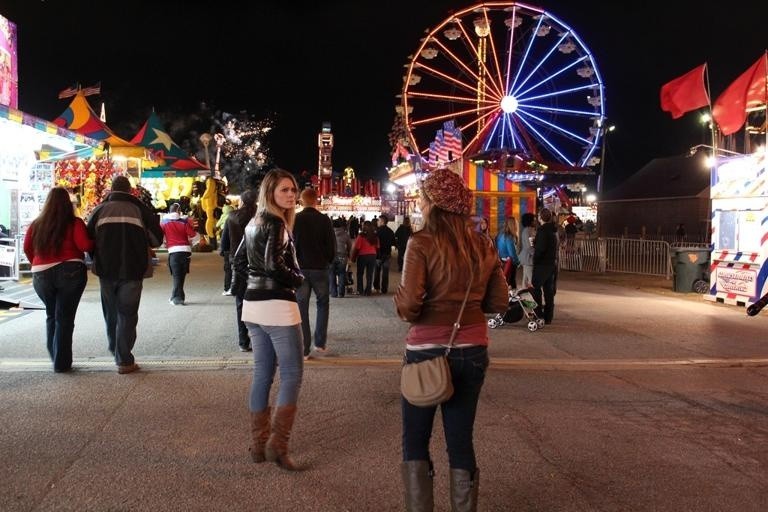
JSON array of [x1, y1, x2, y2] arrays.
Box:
[[424, 170, 473, 215]]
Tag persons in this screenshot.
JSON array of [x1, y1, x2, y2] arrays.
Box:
[[21, 186, 92, 371], [328, 215, 413, 298], [394, 170, 508, 512], [216, 199, 235, 294], [161, 202, 196, 307], [88, 176, 164, 372], [240, 168, 307, 472], [220, 190, 258, 351], [476, 208, 559, 327], [292, 188, 338, 362]]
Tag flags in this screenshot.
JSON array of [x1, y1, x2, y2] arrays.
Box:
[[659, 63, 711, 119], [711, 51, 767, 135]]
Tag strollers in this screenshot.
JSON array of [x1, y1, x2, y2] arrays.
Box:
[[487, 273, 547, 333]]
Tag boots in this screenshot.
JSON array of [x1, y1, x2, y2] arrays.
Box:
[[249, 406, 297, 469]]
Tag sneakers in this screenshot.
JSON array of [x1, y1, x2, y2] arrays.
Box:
[[304, 346, 326, 359], [240, 345, 251, 352], [222, 289, 232, 295], [117, 364, 140, 375]]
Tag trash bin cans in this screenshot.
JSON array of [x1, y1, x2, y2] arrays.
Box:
[[675, 247, 713, 294]]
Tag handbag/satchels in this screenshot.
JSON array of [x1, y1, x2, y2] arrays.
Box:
[[401, 356, 454, 408]]
